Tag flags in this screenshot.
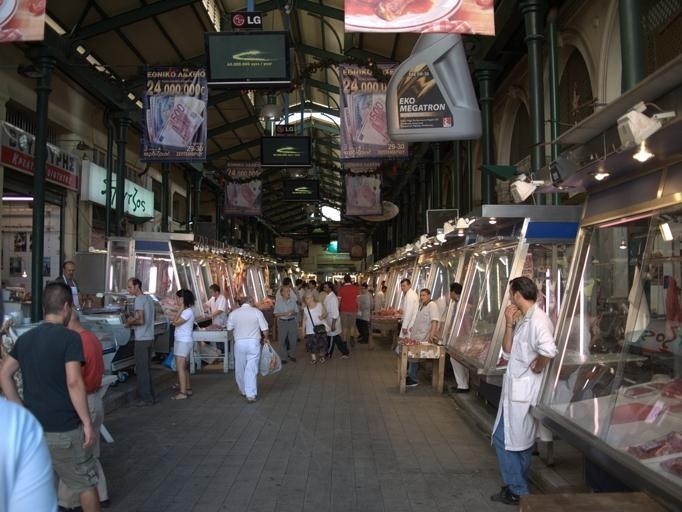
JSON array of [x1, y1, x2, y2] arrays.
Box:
[[145, 67, 208, 163], [225, 162, 263, 216], [275, 235, 293, 256], [337, 228, 362, 251], [345, 167, 384, 216], [338, 61, 412, 161], [0, 0, 46, 44], [296, 237, 308, 257], [345, 0, 496, 37]]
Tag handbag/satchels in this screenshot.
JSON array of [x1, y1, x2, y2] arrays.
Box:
[[314, 324, 329, 348]]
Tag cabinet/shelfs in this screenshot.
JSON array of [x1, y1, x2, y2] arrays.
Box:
[[71, 291, 170, 384]]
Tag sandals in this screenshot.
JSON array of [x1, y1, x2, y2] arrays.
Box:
[[170, 392, 189, 401], [186, 389, 193, 396]]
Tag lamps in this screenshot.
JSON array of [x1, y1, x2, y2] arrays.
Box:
[[587, 159, 613, 181], [629, 136, 655, 164]]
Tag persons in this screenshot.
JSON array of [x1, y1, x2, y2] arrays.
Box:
[[489, 276, 559, 505], [55, 304, 107, 512], [201, 284, 229, 364], [405, 288, 439, 385], [1, 284, 99, 511], [397, 277, 418, 350], [124, 276, 155, 405], [56, 260, 82, 309], [447, 282, 470, 393], [169, 287, 194, 402], [272, 274, 386, 364], [228, 294, 272, 402]]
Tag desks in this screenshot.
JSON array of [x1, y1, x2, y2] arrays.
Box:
[[257, 303, 280, 341], [190, 325, 236, 374], [370, 309, 398, 349], [393, 339, 446, 395], [515, 487, 676, 511]]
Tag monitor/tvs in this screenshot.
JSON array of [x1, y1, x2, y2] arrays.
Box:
[[283, 180, 319, 202], [203, 31, 291, 88], [307, 224, 330, 244], [260, 136, 312, 168]]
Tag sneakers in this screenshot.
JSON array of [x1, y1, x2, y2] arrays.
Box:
[[406, 379, 418, 388], [282, 355, 351, 366]]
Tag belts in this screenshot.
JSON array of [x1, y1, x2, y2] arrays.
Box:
[[280, 317, 295, 321]]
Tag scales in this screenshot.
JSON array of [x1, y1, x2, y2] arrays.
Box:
[[83, 306, 126, 325]]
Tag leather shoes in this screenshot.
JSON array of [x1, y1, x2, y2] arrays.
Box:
[[491, 485, 520, 506]]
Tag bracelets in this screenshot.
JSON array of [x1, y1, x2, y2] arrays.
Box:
[[505, 322, 514, 328]]
